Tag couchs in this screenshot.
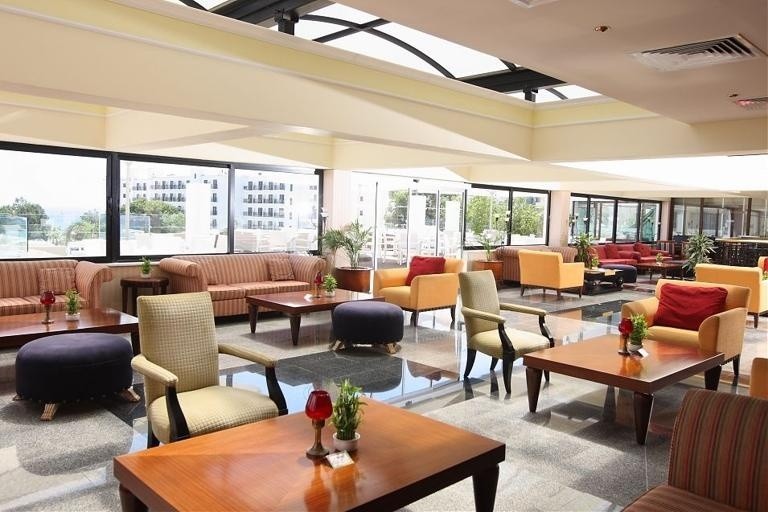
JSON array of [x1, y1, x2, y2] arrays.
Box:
[[471, 229, 768, 301]]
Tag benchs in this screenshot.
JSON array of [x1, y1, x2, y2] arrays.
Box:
[[159, 252, 329, 318], [0, 256, 114, 316]]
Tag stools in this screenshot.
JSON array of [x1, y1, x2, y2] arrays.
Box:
[[328, 300, 406, 355], [11, 331, 141, 419]]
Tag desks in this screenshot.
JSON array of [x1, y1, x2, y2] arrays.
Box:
[[110, 394, 508, 511], [523, 334, 726, 446], [119, 275, 169, 315], [243, 289, 386, 347], [0, 305, 140, 358]]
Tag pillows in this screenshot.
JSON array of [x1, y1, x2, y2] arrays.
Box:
[[266, 258, 295, 282], [653, 281, 729, 333], [36, 267, 77, 295], [191, 258, 219, 285], [404, 255, 446, 287]]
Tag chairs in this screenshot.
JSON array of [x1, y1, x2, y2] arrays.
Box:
[[694, 254, 768, 328], [456, 267, 558, 396], [128, 290, 291, 446], [619, 277, 754, 393], [616, 387, 768, 510], [374, 255, 464, 328]]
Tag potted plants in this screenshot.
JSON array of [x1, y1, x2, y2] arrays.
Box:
[[630, 313, 649, 352], [139, 255, 153, 279], [331, 377, 364, 455], [322, 274, 338, 298], [63, 289, 80, 320], [311, 218, 374, 294]]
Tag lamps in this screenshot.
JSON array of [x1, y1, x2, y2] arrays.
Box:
[[619, 316, 633, 355]]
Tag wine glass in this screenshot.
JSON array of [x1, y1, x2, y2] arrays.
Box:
[[617, 318, 633, 355]]
